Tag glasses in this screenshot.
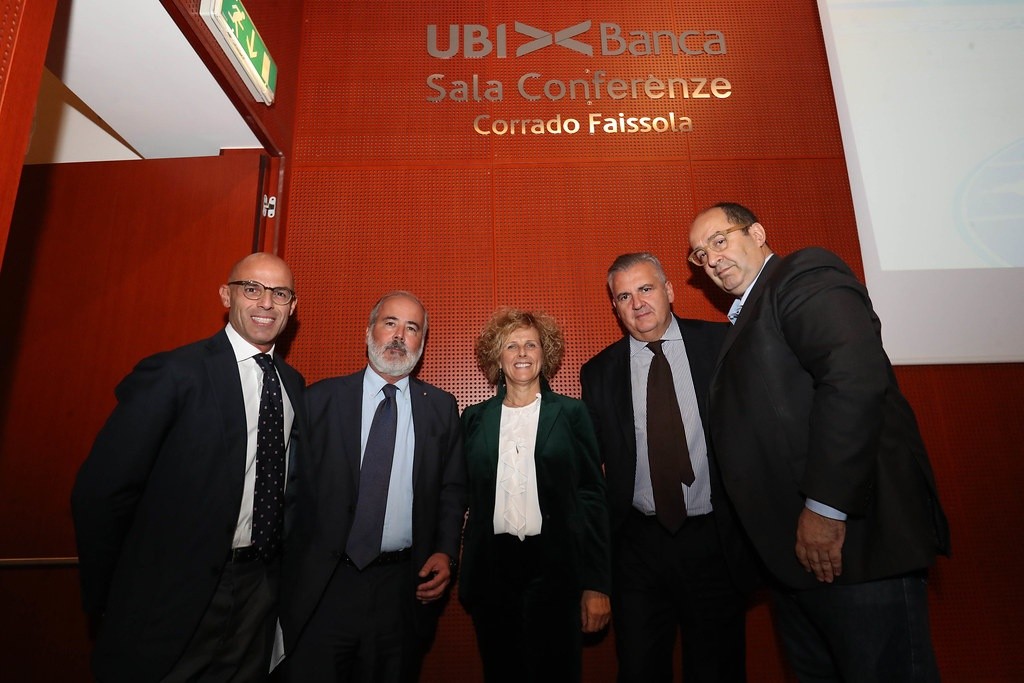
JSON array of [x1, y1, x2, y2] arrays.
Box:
[[688, 222, 750, 266], [227, 280, 297, 306]]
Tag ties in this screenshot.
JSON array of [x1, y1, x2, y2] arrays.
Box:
[[646, 340, 696, 535], [345, 384, 397, 570], [251, 353, 287, 564]]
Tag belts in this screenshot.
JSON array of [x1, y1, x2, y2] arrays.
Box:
[[341, 545, 413, 566], [225, 541, 286, 564]]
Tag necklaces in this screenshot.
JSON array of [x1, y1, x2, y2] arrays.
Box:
[[506, 397, 526, 407]]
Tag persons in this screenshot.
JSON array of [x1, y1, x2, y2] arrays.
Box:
[[280, 291, 467, 683], [461, 308, 611, 683], [70, 252, 305, 683], [688, 201, 951, 683], [579, 252, 769, 683]]
